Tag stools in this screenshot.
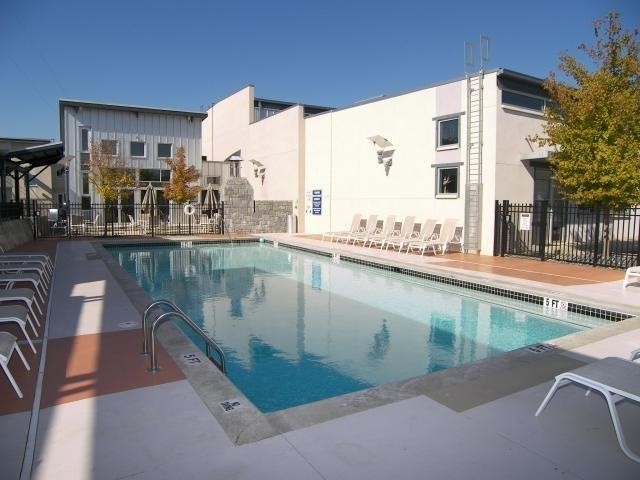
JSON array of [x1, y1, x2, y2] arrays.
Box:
[[535, 347, 639, 466]]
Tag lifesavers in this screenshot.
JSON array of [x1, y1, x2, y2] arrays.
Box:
[[184, 205, 196, 215], [184, 265, 196, 277]]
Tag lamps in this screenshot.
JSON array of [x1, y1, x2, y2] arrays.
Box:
[[248, 157, 262, 179], [368, 134, 393, 166]]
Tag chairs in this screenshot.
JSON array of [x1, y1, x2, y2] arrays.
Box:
[[0, 244, 55, 402], [191, 212, 221, 234], [85, 213, 101, 236], [320, 212, 464, 258], [127, 213, 161, 235]]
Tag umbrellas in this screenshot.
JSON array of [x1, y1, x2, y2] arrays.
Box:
[[139, 181, 160, 237], [202, 182, 219, 219]]
